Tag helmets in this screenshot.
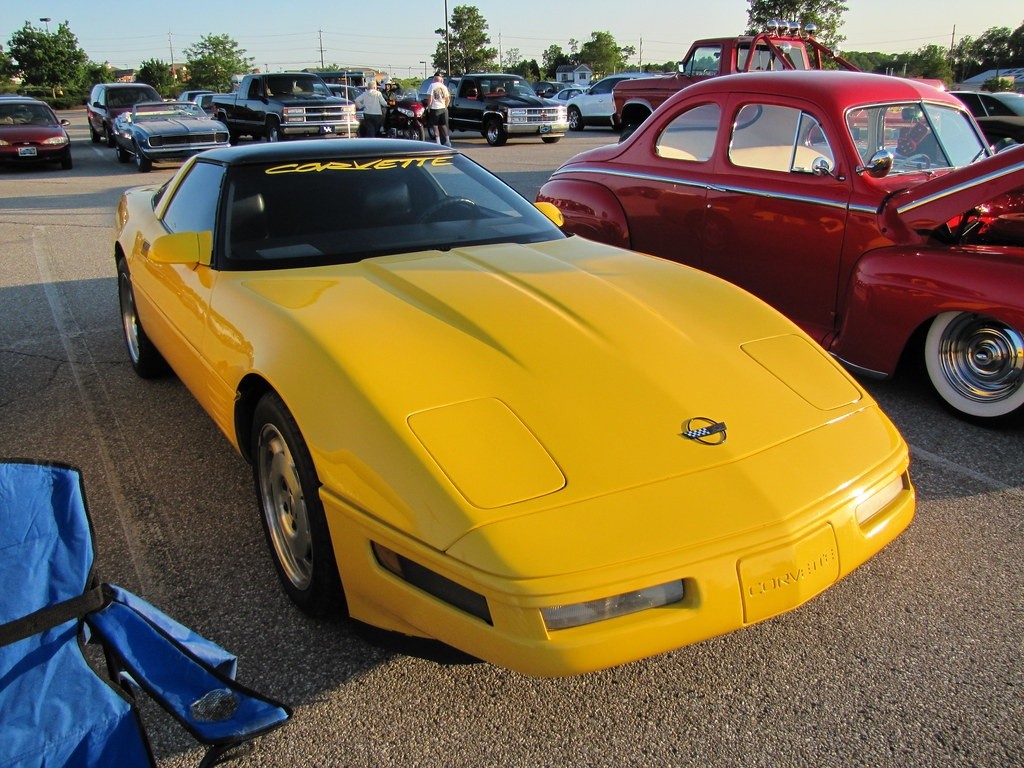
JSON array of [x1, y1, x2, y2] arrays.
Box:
[[380, 77, 393, 90]]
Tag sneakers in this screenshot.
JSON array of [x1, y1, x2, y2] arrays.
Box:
[[443, 142, 451, 147]]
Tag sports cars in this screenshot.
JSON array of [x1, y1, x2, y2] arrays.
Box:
[[114, 139, 917, 681]]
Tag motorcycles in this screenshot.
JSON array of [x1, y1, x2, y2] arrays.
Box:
[[383, 88, 429, 141]]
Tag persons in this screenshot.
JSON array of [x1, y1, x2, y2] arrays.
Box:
[[382, 81, 392, 105], [426, 75, 452, 148], [356, 82, 387, 137]]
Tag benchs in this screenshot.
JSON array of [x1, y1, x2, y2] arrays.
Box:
[[125, 110, 192, 122], [730, 146, 833, 173]]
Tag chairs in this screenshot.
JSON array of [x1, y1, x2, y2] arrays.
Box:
[[496, 86, 505, 92], [292, 85, 302, 93], [0, 456, 294, 768], [0, 112, 13, 125], [231, 188, 267, 241], [352, 179, 411, 225]]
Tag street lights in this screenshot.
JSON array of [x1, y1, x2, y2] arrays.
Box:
[[419, 61, 427, 81]]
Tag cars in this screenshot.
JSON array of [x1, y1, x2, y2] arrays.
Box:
[[535, 69, 1024, 427], [112, 102, 232, 172], [947, 68, 1024, 147], [176, 90, 217, 118], [535, 72, 665, 132], [0, 96, 72, 173]]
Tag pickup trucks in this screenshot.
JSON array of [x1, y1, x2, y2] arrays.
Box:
[[611, 21, 947, 144], [419, 73, 571, 147], [212, 72, 360, 146]]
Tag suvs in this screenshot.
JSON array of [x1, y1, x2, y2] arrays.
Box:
[[87, 83, 175, 148]]
